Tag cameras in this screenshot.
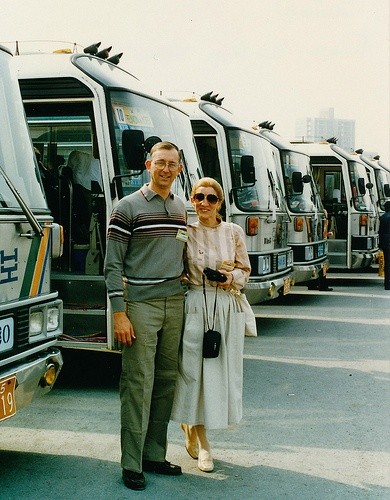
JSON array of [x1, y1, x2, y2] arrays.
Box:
[[203, 267, 228, 283]]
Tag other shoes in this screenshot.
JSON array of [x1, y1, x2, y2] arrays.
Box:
[[197, 443, 214, 471], [319, 287, 333, 291], [308, 286, 319, 290], [385, 286, 390, 290], [180, 423, 199, 459]]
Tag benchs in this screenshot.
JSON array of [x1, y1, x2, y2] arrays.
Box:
[[68, 151, 106, 243]]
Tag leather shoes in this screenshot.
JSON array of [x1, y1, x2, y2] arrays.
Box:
[[122, 468, 146, 491], [142, 460, 182, 475]]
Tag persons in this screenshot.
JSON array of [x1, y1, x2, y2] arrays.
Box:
[[170, 177, 251, 472], [103, 141, 188, 491], [378, 201, 390, 290], [33, 147, 65, 224]]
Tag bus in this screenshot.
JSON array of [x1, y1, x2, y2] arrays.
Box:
[[0, 40, 67, 425], [161, 91, 288, 315], [4, 43, 212, 399], [290, 129, 390, 276], [253, 123, 329, 293]]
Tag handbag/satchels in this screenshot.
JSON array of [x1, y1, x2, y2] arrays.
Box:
[[236, 289, 257, 337]]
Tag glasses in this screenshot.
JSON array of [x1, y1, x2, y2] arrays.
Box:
[[192, 193, 221, 205], [151, 160, 179, 171]]
